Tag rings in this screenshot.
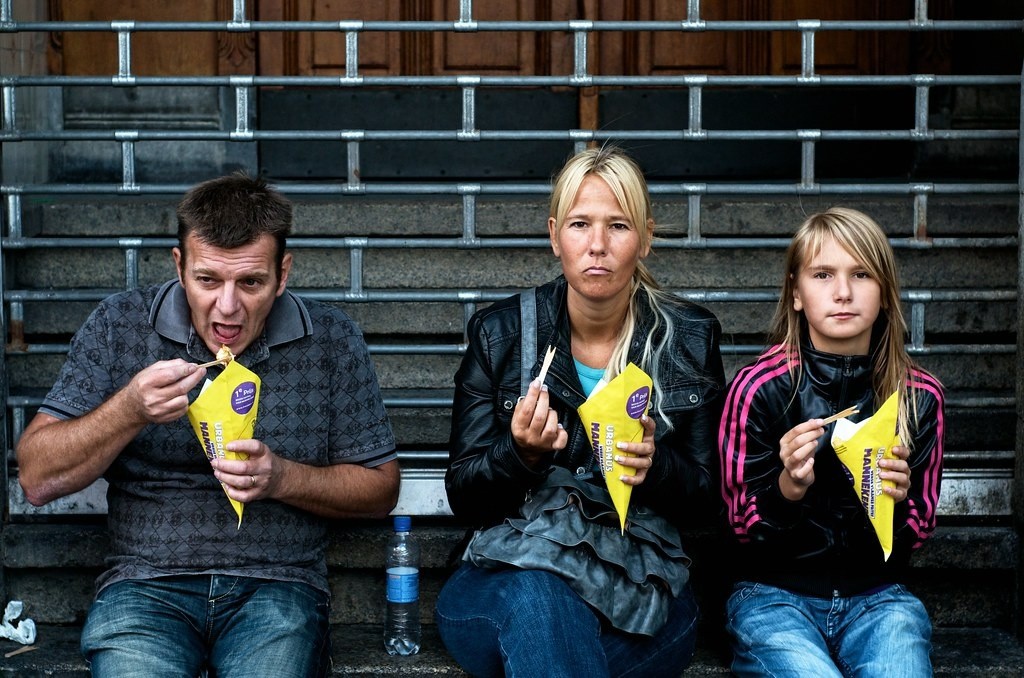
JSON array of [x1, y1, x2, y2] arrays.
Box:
[[642, 456, 652, 470], [249, 473, 256, 490]]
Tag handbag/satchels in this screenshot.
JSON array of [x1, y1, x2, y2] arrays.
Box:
[[460, 463, 692, 641]]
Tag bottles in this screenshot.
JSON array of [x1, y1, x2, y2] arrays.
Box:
[[381, 514, 422, 657]]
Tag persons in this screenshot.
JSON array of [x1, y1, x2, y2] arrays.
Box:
[[710, 204, 947, 677], [13, 178, 403, 678], [427, 149, 726, 678]]
